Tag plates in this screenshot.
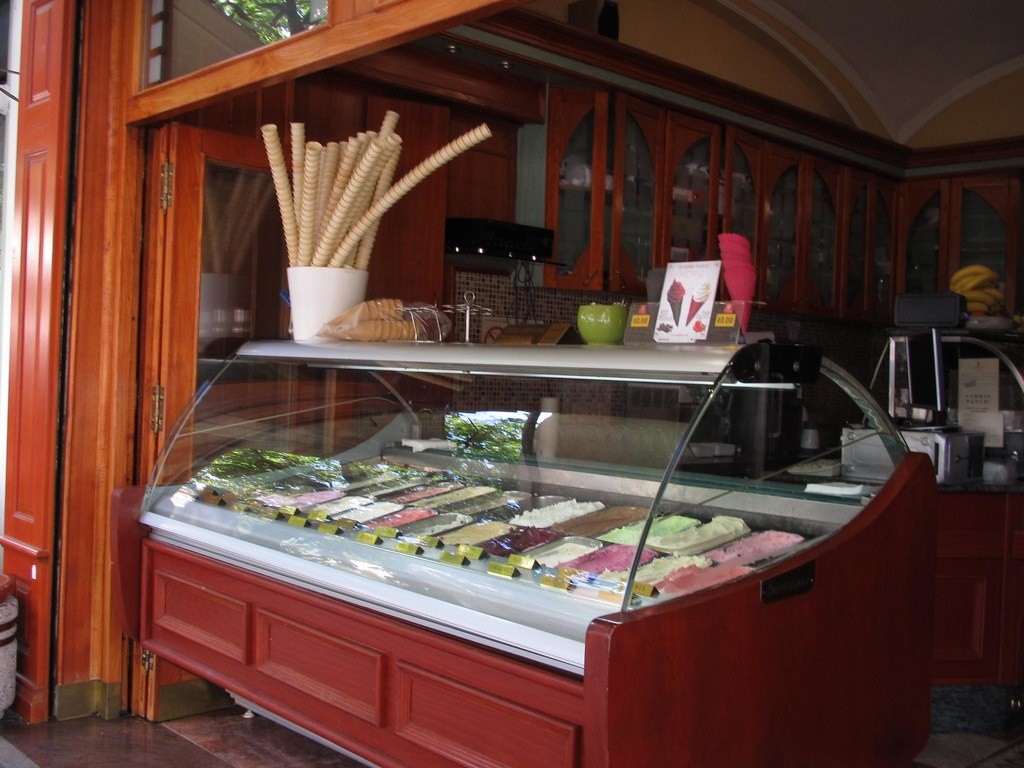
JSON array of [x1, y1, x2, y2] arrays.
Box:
[[964, 316, 1014, 331]]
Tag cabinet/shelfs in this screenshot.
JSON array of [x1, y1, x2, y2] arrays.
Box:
[[840, 165, 900, 323], [660, 111, 765, 308], [299, 46, 547, 340], [896, 172, 1024, 317], [516, 83, 667, 296], [759, 141, 844, 319]]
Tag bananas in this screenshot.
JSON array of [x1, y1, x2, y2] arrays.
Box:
[[951, 264, 1004, 316]]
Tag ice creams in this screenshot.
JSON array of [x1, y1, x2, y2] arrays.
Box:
[[686, 281, 712, 326], [667, 279, 685, 327]]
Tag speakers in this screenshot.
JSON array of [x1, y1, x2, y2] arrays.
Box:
[[568, 0, 620, 42]]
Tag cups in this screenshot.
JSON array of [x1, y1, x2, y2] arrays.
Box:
[[578, 305, 627, 345], [286, 266, 369, 340], [799, 429, 819, 449]]
